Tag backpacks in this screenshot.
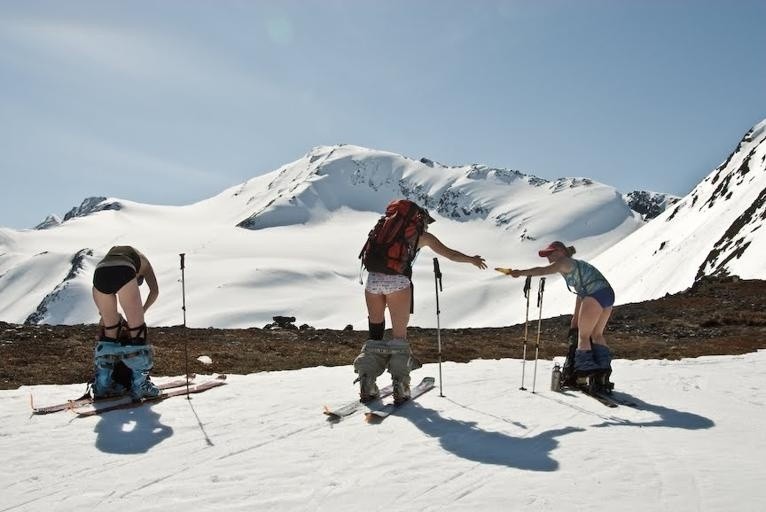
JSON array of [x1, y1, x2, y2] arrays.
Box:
[[362, 198, 422, 276]]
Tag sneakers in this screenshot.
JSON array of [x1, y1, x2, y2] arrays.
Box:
[[571, 374, 598, 385], [359, 382, 380, 395], [130, 381, 159, 396], [599, 373, 609, 384], [392, 385, 412, 399], [91, 381, 126, 394]]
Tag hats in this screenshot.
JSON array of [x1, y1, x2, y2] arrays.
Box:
[[538, 241, 569, 258], [420, 207, 437, 224]]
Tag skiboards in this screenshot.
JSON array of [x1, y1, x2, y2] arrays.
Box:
[[578, 382, 639, 408], [29, 373, 228, 416], [323, 376, 435, 418]]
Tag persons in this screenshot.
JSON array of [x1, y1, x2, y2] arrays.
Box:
[[504, 239, 615, 394], [357, 201, 488, 406], [89, 244, 164, 398]]
